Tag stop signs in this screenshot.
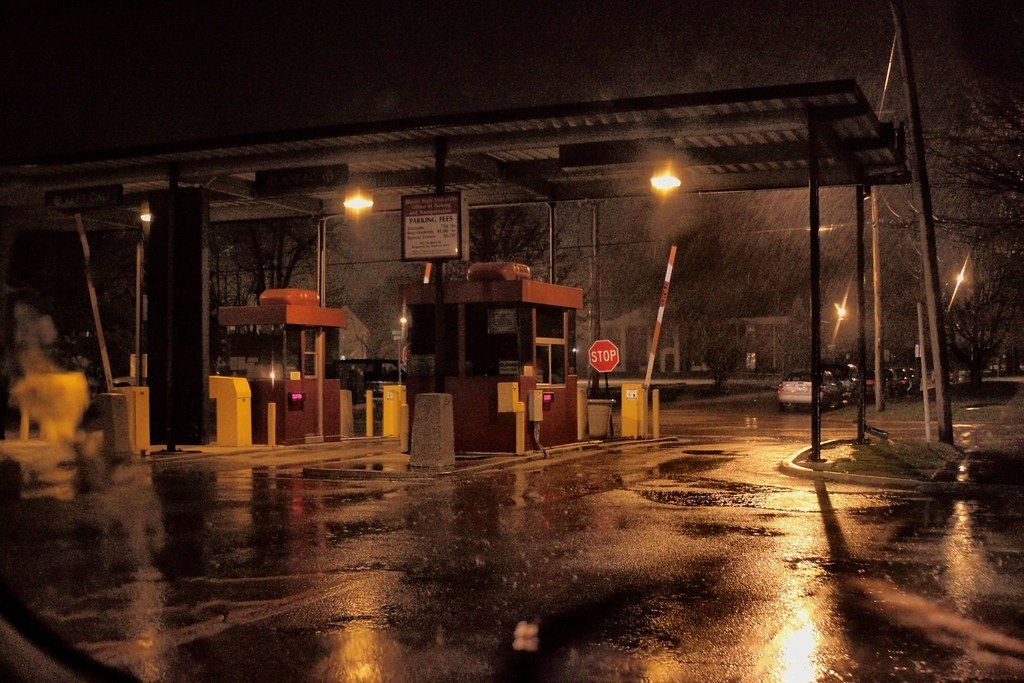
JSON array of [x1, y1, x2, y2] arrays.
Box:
[[588, 339, 620, 373]]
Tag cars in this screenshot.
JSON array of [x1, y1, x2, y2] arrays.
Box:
[[777, 369, 841, 413], [824, 363, 920, 405], [335, 357, 407, 398]]
